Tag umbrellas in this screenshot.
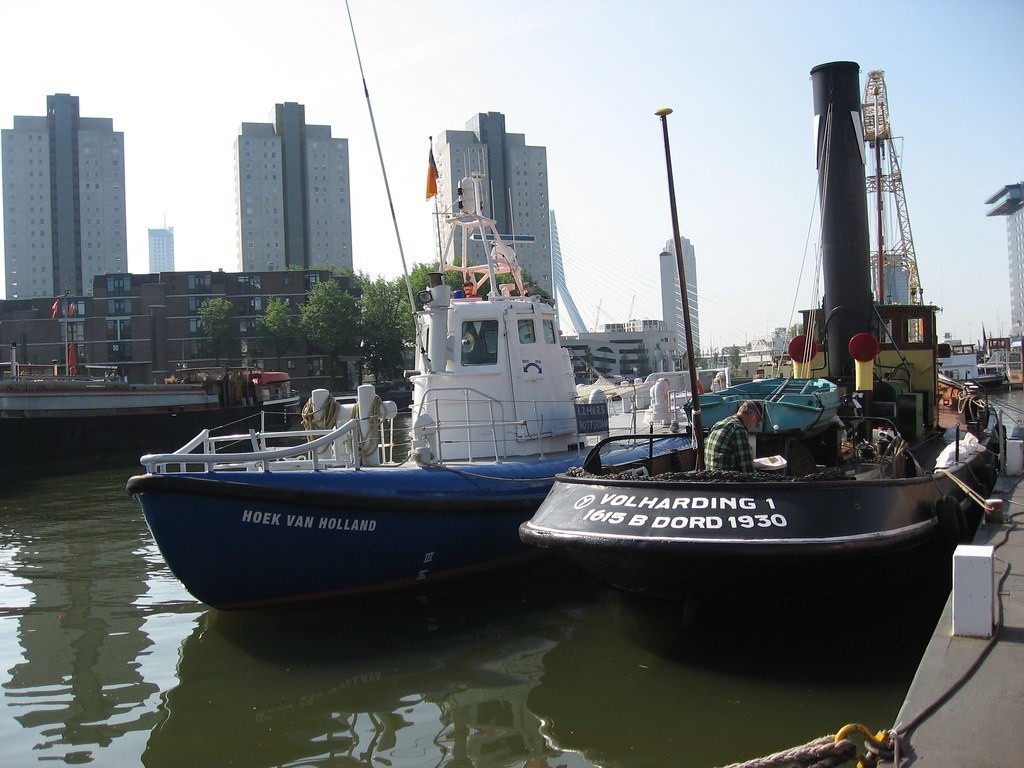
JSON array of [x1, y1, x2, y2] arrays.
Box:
[[69, 342, 76, 376]]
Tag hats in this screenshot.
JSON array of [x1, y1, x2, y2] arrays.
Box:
[[743, 400, 765, 423]]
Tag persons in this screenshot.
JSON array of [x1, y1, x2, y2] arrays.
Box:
[[704, 400, 765, 473], [104, 370, 119, 381]]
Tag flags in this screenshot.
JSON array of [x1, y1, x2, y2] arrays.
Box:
[[425, 147, 439, 202], [68, 299, 75, 315], [51, 300, 59, 318]]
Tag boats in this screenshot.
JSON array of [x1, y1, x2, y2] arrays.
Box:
[[126, 174, 693, 613], [682, 377, 841, 435], [519, 61, 1007, 604], [938, 338, 1024, 388]]
